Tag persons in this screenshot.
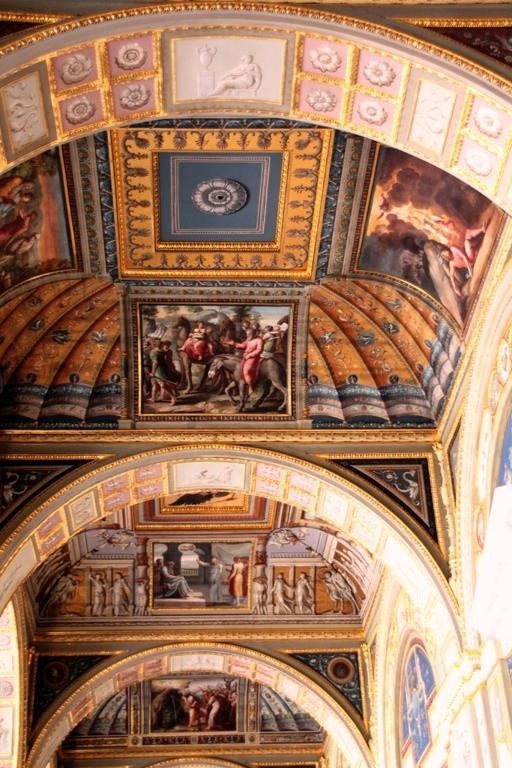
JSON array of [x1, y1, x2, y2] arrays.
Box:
[[160, 560, 193, 598], [440, 218, 488, 299], [195, 556, 224, 603], [269, 571, 294, 616], [225, 555, 244, 605], [331, 568, 361, 615], [87, 572, 107, 617], [0, 174, 35, 205], [206, 51, 263, 99], [138, 311, 290, 406], [292, 571, 314, 615], [0, 231, 42, 265], [108, 571, 130, 615], [321, 571, 343, 611], [394, 236, 425, 288], [148, 682, 236, 732], [0, 192, 33, 218], [0, 206, 39, 248]]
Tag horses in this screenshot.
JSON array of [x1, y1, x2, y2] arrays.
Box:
[[142, 314, 290, 411]]
